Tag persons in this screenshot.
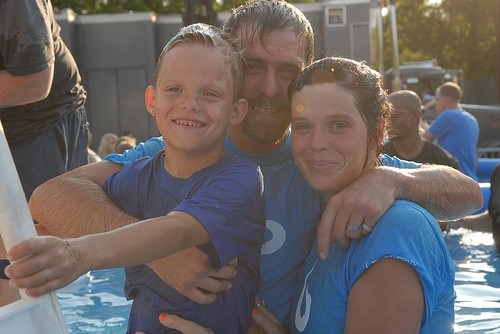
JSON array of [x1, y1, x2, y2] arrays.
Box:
[[134, 56, 457, 333], [28, 0, 485, 334], [420, 81, 480, 182], [0, 0, 90, 201], [446, 162, 500, 255], [380, 90, 459, 169], [0, 21, 265, 334]]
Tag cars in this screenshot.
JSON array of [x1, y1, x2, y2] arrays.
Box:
[[384, 63, 452, 101]]
